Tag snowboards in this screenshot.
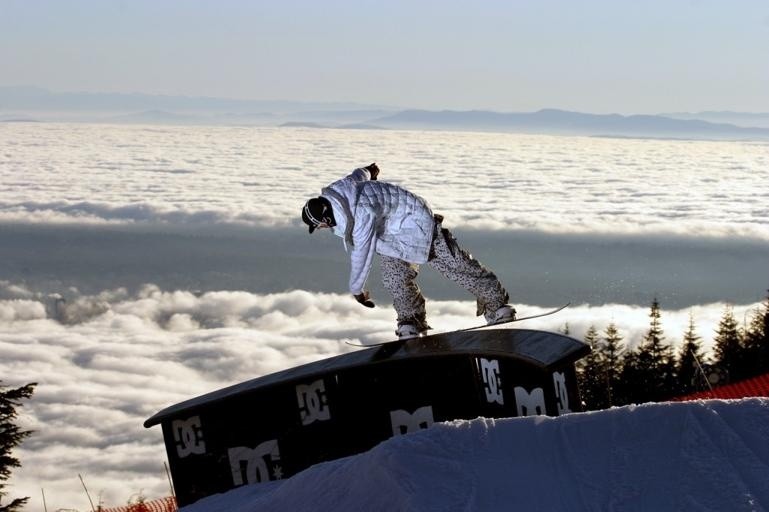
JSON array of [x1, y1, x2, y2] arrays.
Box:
[[345, 299, 570, 346]]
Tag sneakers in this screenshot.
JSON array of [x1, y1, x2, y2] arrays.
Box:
[[483, 302, 516, 326], [394, 320, 432, 341]]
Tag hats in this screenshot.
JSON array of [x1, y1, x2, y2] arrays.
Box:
[[301, 196, 336, 234]]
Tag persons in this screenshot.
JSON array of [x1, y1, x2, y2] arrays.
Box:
[[303, 164, 515, 341]]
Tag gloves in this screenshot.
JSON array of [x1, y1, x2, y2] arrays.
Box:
[[353, 290, 376, 308], [364, 163, 380, 180]]
